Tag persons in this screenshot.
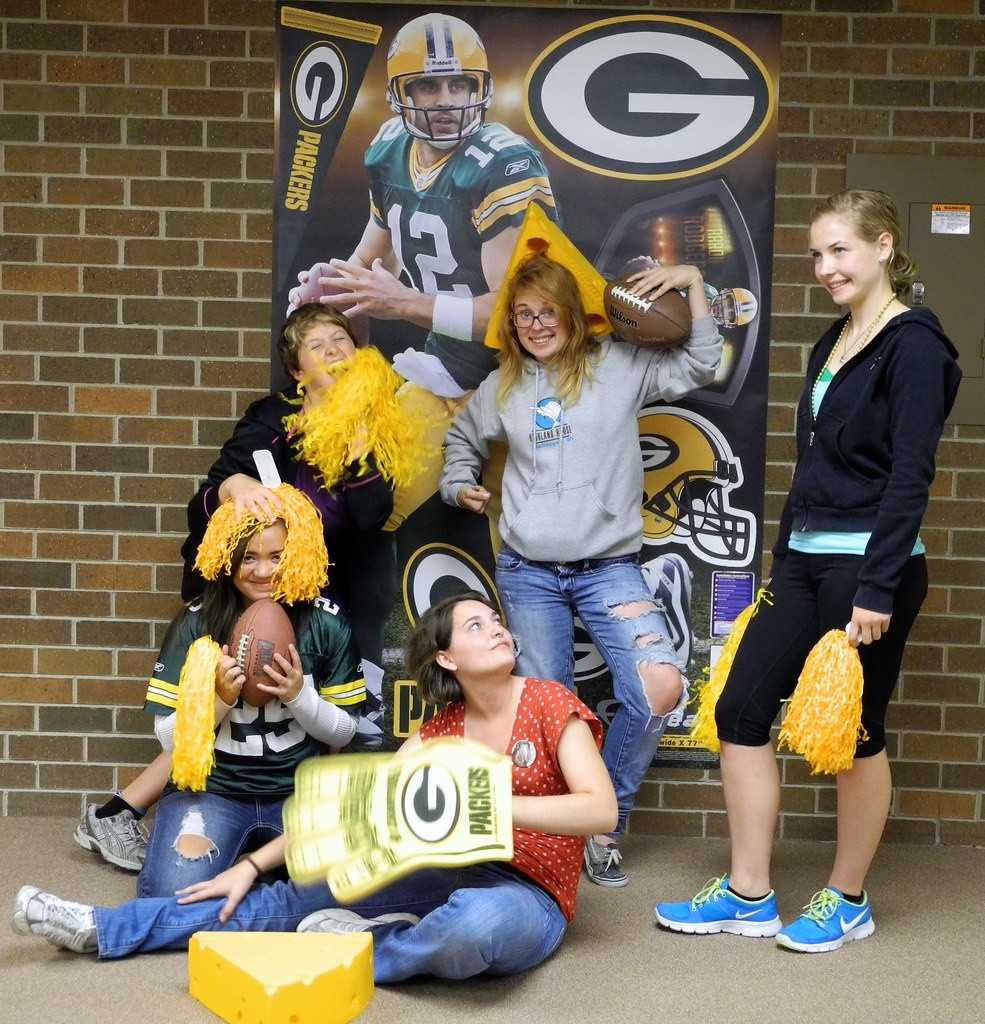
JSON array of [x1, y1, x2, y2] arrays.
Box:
[[439, 252, 724, 885], [10, 591, 622, 986], [285, 11, 564, 751], [134, 484, 367, 905], [70, 301, 398, 877], [619, 253, 760, 329], [651, 184, 962, 957]]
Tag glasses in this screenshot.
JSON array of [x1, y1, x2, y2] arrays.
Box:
[[509, 310, 561, 327]]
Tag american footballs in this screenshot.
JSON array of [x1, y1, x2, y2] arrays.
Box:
[[295, 261, 368, 352], [228, 598, 296, 709], [603, 274, 693, 349]]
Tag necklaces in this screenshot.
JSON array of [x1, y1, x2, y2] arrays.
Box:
[[810, 289, 899, 424], [838, 320, 874, 364]]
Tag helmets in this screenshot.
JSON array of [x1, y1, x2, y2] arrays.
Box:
[[709, 288, 758, 329], [386, 12, 493, 149]]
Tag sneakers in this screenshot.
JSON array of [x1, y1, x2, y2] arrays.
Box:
[[655, 876, 782, 938], [296, 908, 421, 935], [582, 835, 627, 887], [10, 885, 97, 953], [774, 886, 874, 953], [73, 803, 149, 872], [640, 552, 698, 673]]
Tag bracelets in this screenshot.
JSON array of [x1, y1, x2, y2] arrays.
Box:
[[246, 857, 266, 879]]
